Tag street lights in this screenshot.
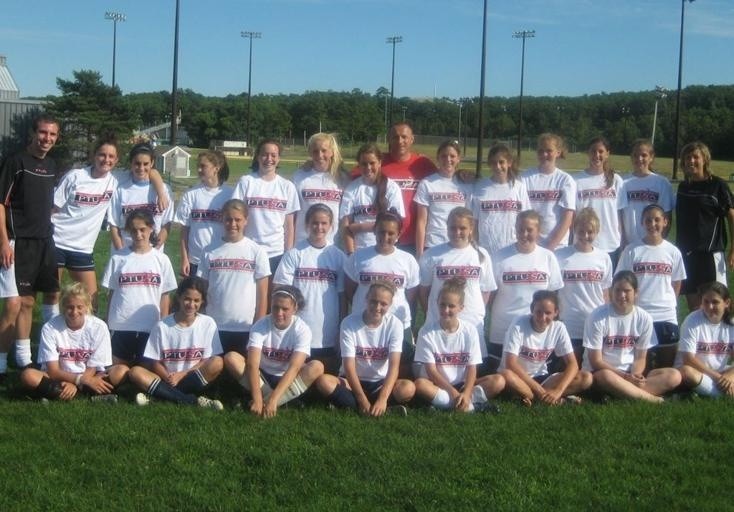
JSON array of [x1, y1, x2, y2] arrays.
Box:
[[386, 36, 402, 125], [240, 30, 262, 147], [557, 106, 564, 135], [652, 85, 671, 145], [105, 11, 125, 88], [512, 29, 536, 157]]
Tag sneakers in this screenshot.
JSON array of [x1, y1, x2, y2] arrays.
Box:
[[385, 406, 408, 418], [16, 360, 41, 371], [198, 395, 223, 410], [288, 399, 304, 408], [325, 402, 336, 411], [665, 389, 697, 404], [565, 395, 582, 404], [601, 394, 614, 403], [472, 402, 499, 415], [135, 393, 153, 406], [90, 394, 118, 405], [523, 397, 534, 405], [0, 373, 8, 391]]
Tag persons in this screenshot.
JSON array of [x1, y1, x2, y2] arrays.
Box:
[[672, 281, 734, 401], [130, 122, 685, 419], [0, 112, 179, 404], [675, 140, 734, 313]]
